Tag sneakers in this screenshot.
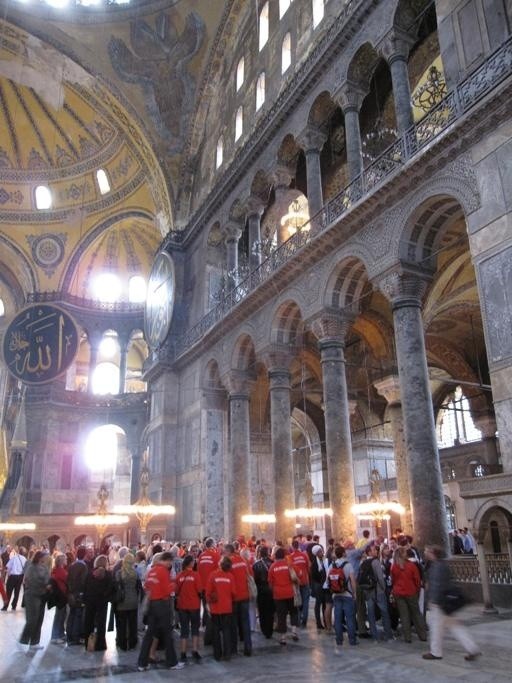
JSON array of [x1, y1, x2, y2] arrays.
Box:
[[423, 653, 442, 660], [51, 634, 67, 644], [180, 651, 202, 660], [19, 643, 44, 649], [169, 662, 185, 670], [138, 664, 151, 672], [464, 652, 483, 661]]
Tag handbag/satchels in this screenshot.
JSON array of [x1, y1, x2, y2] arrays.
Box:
[[433, 580, 467, 615]]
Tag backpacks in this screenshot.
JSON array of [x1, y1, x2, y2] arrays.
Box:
[[358, 556, 377, 589], [327, 561, 348, 594]]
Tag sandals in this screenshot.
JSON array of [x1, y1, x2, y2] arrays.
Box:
[[280, 638, 286, 645], [292, 634, 298, 640]]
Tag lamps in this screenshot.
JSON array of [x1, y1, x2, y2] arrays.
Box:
[[116, 380, 175, 533], [238, 332, 279, 532], [73, 391, 131, 541], [348, 327, 403, 529], [2, 387, 36, 539], [285, 328, 333, 530]]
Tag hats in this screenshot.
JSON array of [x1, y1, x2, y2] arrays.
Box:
[[311, 545, 322, 556]]
